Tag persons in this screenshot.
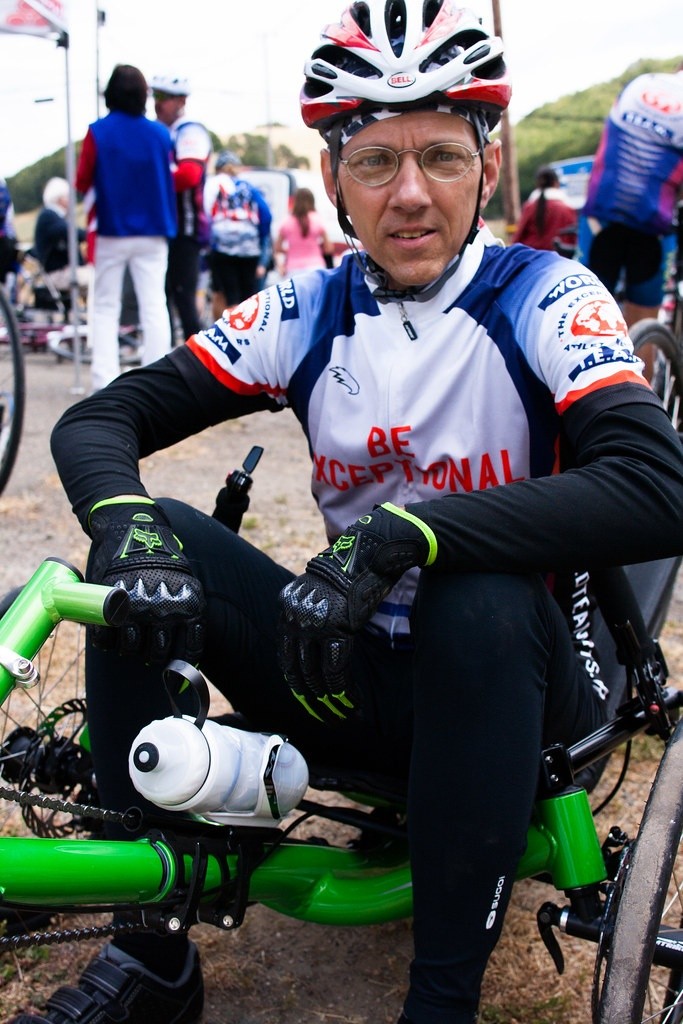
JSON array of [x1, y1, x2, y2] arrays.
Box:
[[14, 0, 683, 1023], [1, 58, 682, 402]]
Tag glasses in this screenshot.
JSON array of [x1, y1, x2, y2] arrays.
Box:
[[338, 142, 480, 189]]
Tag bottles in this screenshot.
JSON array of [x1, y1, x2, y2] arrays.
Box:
[[127, 657, 308, 812]]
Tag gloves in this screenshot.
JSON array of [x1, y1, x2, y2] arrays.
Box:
[[86, 495, 211, 693], [275, 497, 439, 723]]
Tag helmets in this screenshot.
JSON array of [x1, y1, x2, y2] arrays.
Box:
[[299, 0, 513, 135], [147, 72, 192, 97]]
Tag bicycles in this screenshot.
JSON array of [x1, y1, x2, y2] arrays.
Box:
[[626, 204, 682, 441], [1, 283, 147, 497], [0, 446, 683, 1023]]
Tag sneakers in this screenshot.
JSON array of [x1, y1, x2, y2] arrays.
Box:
[[8, 937, 207, 1024]]
